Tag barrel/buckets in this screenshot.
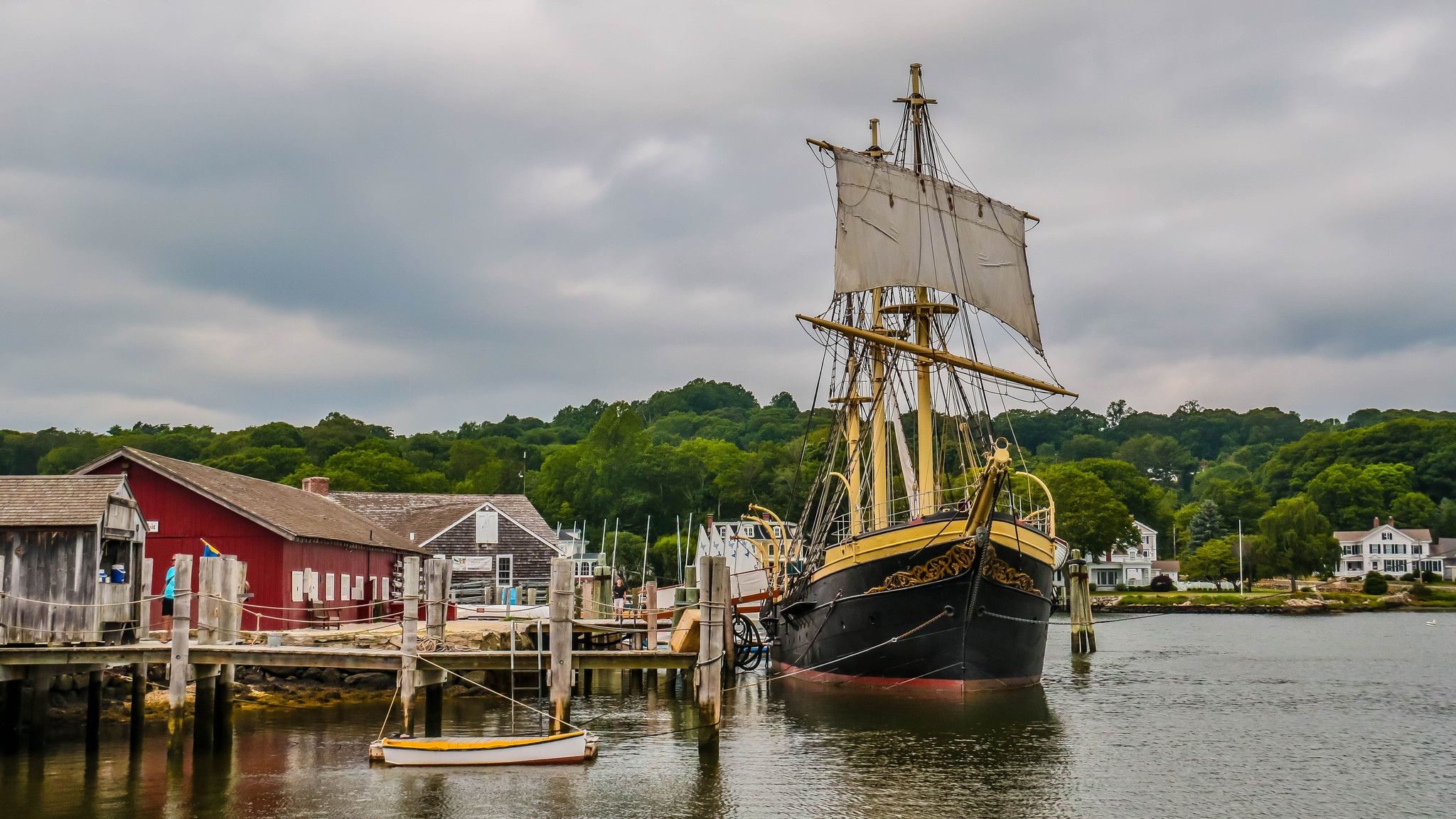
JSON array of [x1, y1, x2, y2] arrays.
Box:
[[99, 563, 126, 583]]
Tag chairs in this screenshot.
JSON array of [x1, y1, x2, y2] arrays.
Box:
[[311, 598, 343, 630]]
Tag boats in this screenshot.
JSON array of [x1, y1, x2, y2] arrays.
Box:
[[367, 728, 595, 769]]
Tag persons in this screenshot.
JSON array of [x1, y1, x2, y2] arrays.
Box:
[[156, 553, 183, 644], [613, 579, 627, 621]]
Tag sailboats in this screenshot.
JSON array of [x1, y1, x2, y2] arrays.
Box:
[[754, 59, 1088, 703]]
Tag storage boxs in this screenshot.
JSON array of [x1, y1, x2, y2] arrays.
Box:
[[669, 609, 700, 653]]
